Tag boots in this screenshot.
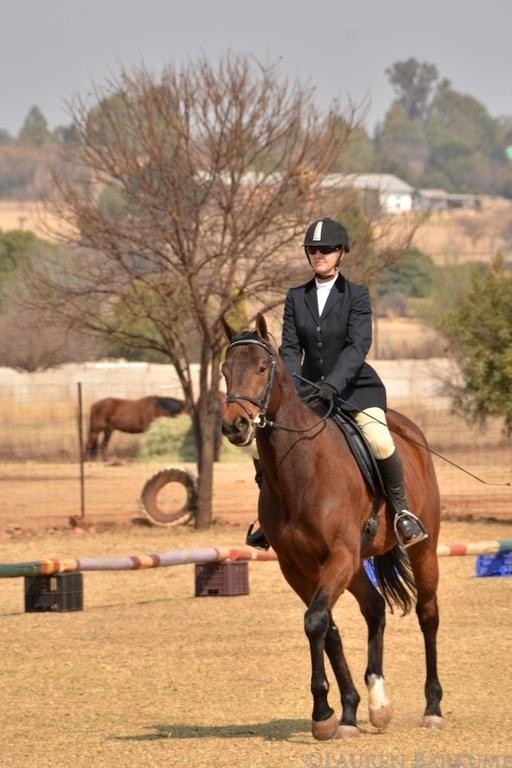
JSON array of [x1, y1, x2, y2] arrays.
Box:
[[373, 445, 425, 544], [246, 526, 271, 549]]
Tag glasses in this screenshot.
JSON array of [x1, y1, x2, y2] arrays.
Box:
[[305, 245, 341, 255]]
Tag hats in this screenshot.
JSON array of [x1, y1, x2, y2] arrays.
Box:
[[300, 216, 350, 253]]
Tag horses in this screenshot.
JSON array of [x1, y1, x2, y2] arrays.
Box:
[[82, 396, 191, 465], [218, 310, 446, 742]]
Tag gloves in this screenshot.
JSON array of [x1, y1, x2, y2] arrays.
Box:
[[317, 381, 339, 404]]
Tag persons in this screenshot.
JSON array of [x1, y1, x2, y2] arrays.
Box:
[[245, 216, 422, 547]]
